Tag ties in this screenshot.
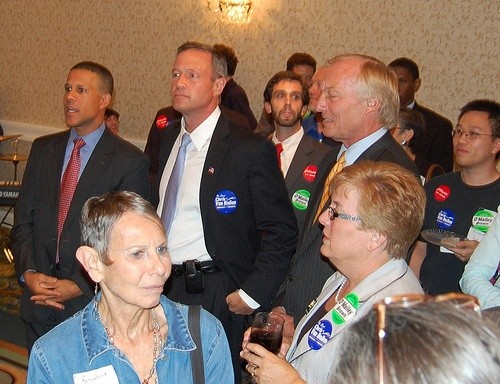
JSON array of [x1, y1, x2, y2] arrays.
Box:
[[55, 138, 85, 264], [275, 143, 283, 169], [161, 133, 193, 239], [311, 152, 348, 227]]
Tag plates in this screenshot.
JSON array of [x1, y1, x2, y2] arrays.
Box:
[[421, 229, 470, 247]]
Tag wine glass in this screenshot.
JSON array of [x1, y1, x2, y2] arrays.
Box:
[[242, 312, 285, 384]]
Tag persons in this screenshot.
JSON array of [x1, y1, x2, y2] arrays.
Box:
[[26, 191, 235, 384], [104, 41, 500, 384], [240, 159, 426, 384], [12, 61, 150, 357]]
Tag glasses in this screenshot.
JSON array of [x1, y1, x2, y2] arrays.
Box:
[[328, 207, 363, 224], [451, 130, 498, 139], [373, 293, 483, 384]]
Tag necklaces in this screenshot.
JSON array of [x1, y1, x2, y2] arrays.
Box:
[[335, 277, 347, 303], [97, 307, 162, 384]]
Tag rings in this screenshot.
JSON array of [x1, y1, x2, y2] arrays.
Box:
[[252, 366, 259, 377]]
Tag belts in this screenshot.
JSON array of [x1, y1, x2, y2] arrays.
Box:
[[169, 264, 218, 277]]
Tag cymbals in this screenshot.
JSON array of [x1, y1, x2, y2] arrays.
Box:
[[0, 134, 22, 141], [0, 154, 29, 161]]
